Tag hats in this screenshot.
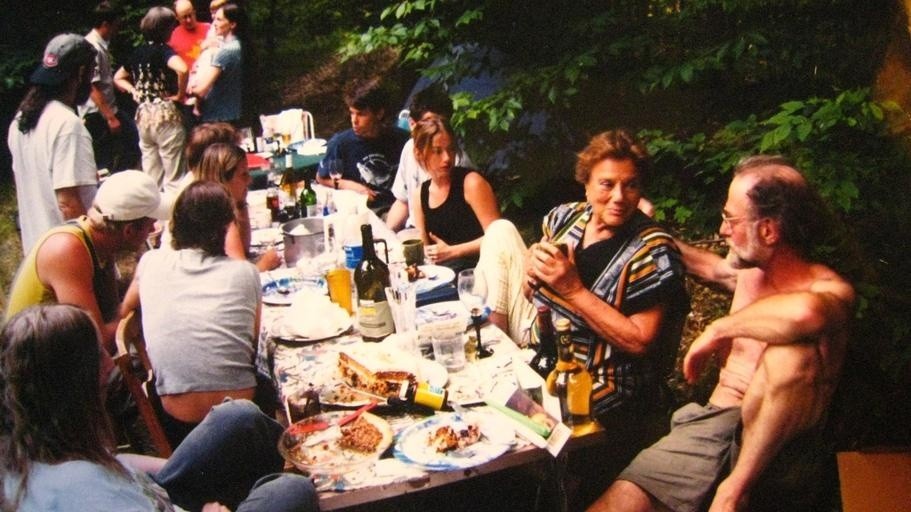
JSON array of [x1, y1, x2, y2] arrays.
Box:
[[90, 168, 178, 225], [30, 32, 98, 84]]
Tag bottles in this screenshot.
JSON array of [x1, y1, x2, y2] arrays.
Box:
[[265, 151, 317, 222], [547, 318, 593, 435], [400, 380, 449, 411], [529, 307, 558, 379], [355, 225, 396, 344]]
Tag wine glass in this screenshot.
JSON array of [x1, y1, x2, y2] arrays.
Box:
[[329, 158, 343, 192], [460, 269, 495, 360]]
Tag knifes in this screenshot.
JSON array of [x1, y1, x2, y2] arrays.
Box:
[[301, 403, 377, 446]]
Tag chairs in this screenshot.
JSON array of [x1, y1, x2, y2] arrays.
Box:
[[113, 305, 179, 458]]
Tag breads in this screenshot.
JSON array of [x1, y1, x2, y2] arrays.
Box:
[[337, 353, 415, 401], [405, 262, 425, 280]]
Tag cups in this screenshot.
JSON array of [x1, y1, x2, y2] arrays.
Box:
[[255, 209, 271, 229], [282, 134, 291, 148], [329, 271, 353, 315], [401, 240, 426, 264], [429, 334, 467, 374], [345, 243, 363, 268]]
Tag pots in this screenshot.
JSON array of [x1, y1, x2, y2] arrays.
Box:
[[280, 217, 337, 269]]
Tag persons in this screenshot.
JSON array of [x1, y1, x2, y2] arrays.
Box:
[[589, 158, 860, 512], [0, 0, 686, 511]]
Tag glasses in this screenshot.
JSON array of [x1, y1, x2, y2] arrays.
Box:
[[721, 212, 754, 227]]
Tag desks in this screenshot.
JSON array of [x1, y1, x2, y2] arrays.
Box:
[[836, 448, 910, 512]]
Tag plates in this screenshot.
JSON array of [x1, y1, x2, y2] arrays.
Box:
[[408, 301, 493, 348], [270, 304, 353, 343], [278, 410, 392, 477], [260, 277, 331, 306], [288, 139, 328, 151], [330, 355, 449, 400], [399, 263, 456, 296], [250, 229, 285, 246], [394, 412, 516, 472]]
